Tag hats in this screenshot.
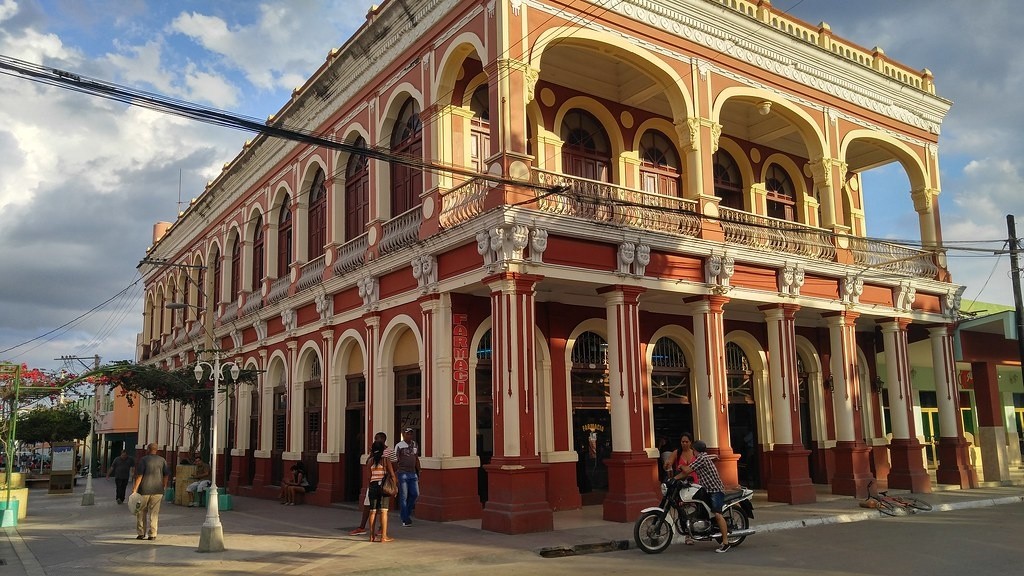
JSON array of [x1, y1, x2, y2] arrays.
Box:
[[403, 427, 413, 433]]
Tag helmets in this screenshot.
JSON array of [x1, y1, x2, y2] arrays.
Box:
[[691, 440, 706, 452]]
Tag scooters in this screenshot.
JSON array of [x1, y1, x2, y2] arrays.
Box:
[[82, 459, 101, 478]]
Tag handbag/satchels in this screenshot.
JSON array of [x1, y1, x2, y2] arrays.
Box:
[[382, 473, 400, 500]]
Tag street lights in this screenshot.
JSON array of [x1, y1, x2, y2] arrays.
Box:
[[164, 303, 215, 508], [191, 349, 240, 554]]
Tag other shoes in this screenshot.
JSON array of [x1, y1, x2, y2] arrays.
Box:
[[370, 536, 377, 542], [136, 535, 144, 539], [148, 537, 155, 540], [117, 498, 121, 504], [380, 537, 395, 542], [188, 502, 202, 507], [400, 518, 412, 526], [284, 501, 296, 506], [684, 536, 694, 545], [281, 500, 288, 504]]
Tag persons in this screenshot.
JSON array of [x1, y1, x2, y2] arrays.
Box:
[[365, 441, 396, 542], [659, 431, 677, 481], [664, 433, 697, 545], [14, 453, 51, 469], [675, 440, 730, 553], [132, 443, 168, 539], [0, 454, 3, 467], [281, 461, 311, 505], [186, 456, 212, 507], [394, 427, 420, 527], [348, 432, 393, 536], [744, 428, 754, 448], [106, 450, 135, 505]]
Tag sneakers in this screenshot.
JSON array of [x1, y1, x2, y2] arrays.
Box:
[[348, 527, 367, 536], [375, 529, 382, 536], [715, 541, 731, 552]]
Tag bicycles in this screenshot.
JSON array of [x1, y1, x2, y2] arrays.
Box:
[[862, 482, 933, 516]]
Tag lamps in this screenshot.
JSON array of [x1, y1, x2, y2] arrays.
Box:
[[872, 376, 884, 394], [166, 303, 206, 311], [824, 375, 834, 393]]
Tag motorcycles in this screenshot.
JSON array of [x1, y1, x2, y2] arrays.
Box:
[[634, 470, 756, 554]]
